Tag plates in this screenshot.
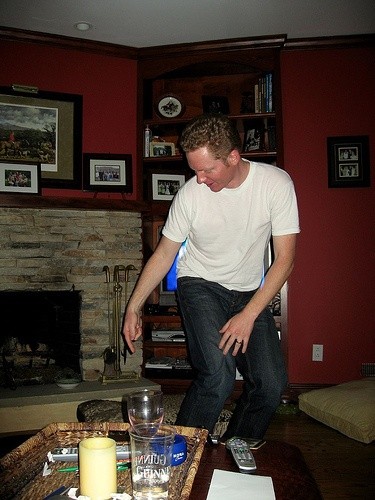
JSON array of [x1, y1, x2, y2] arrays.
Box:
[[155, 94, 186, 120]]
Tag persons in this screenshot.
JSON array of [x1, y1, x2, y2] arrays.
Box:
[[7, 171, 28, 187], [98, 168, 119, 182], [208, 99, 224, 112], [158, 181, 180, 195], [153, 146, 171, 157], [122, 111, 301, 445], [340, 149, 356, 160], [6, 130, 17, 150], [340, 165, 357, 176]]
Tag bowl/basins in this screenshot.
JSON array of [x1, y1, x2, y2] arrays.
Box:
[[151, 435, 187, 467], [56, 380, 81, 390]]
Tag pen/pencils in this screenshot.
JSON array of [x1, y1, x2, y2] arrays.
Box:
[[60, 484, 73, 496], [56, 461, 129, 476]]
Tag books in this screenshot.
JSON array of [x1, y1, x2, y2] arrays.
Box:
[[254, 73, 273, 113]]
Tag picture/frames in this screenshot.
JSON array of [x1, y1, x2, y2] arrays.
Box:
[[327, 136, 370, 188], [83, 153, 134, 193], [150, 142, 176, 157], [0, 160, 42, 196], [0, 84, 82, 190], [154, 93, 185, 120], [202, 95, 230, 115], [152, 174, 186, 201]]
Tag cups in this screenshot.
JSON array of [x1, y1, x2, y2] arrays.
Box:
[[79, 437, 117, 500], [128, 423, 176, 500]]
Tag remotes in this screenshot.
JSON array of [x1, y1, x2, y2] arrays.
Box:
[[230, 438, 258, 474], [52, 444, 143, 462]]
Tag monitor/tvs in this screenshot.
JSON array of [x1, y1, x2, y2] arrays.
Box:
[[159, 224, 271, 305]]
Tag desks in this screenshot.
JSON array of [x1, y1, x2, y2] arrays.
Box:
[[189, 438, 323, 500]]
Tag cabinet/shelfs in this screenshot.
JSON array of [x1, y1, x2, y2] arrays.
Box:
[[138, 34, 287, 213], [142, 280, 289, 402]]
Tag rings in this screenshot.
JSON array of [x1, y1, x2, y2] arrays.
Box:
[[236, 340, 242, 344]]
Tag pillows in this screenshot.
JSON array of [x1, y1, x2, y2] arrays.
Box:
[[298, 377, 374, 444], [77, 394, 233, 438]]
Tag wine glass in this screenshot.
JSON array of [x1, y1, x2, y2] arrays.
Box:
[[126, 390, 165, 454]]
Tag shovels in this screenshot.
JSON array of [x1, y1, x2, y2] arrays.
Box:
[[102, 265, 116, 364]]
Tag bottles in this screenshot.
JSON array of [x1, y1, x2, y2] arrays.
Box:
[[152, 136, 160, 142], [145, 125, 152, 158]]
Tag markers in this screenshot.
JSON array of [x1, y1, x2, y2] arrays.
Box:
[[43, 486, 65, 500]]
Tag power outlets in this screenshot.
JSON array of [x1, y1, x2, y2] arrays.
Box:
[[311, 344, 323, 362]]
